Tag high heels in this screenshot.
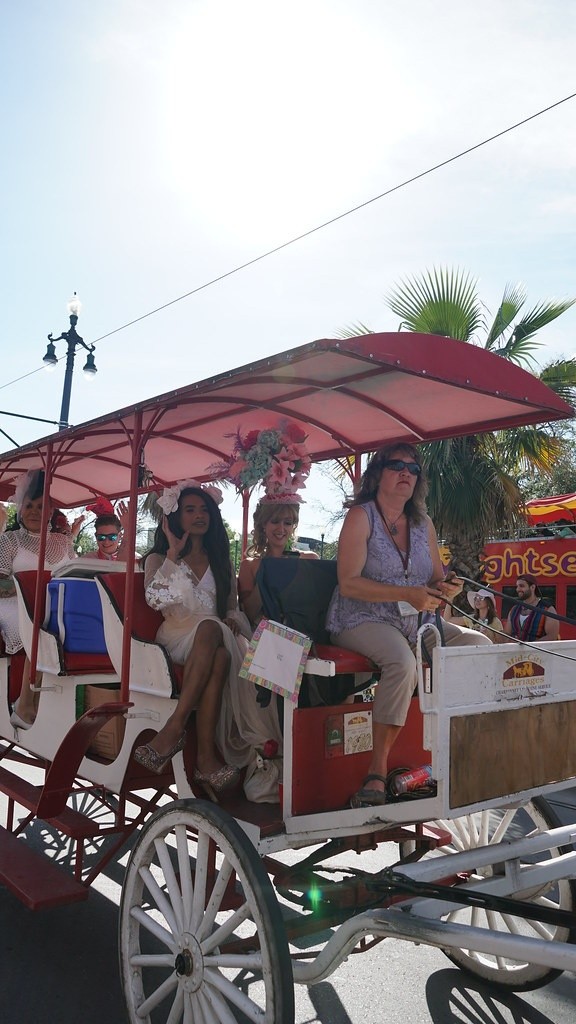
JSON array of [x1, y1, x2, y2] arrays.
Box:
[[192, 765, 239, 804], [133, 731, 189, 776]]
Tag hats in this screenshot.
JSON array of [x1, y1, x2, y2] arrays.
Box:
[[472, 588, 496, 608], [517, 574, 542, 597]]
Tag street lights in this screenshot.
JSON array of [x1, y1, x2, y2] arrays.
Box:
[[41, 291, 97, 431], [320, 526, 326, 560], [234, 532, 240, 571]]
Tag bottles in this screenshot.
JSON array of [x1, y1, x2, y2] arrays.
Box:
[[394, 763, 435, 794]]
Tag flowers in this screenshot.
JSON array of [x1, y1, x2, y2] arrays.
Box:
[[201, 417, 313, 502], [157, 478, 223, 516]]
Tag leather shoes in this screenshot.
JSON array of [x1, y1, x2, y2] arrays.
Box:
[[10, 712, 32, 730]]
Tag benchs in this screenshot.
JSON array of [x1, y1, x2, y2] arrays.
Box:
[[12, 568, 118, 678], [93, 571, 182, 700], [254, 556, 430, 677]]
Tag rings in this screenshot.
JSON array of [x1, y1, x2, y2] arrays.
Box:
[[427, 610, 432, 614]]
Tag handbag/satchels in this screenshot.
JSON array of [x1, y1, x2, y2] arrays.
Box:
[[387, 766, 436, 803], [243, 754, 283, 803]]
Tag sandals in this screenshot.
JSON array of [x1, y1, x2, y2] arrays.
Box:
[[354, 774, 386, 808]]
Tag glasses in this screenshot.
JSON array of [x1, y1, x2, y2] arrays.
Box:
[[473, 595, 485, 600], [94, 530, 121, 541], [384, 460, 421, 475]]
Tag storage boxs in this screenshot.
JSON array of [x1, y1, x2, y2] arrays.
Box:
[[85, 684, 125, 759]]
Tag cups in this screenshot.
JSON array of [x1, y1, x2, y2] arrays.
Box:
[[480, 621, 487, 633], [277, 780, 283, 812]]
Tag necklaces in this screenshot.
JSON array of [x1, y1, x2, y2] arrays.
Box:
[[383, 512, 404, 535]]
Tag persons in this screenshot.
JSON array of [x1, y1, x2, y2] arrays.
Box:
[[444, 590, 503, 645], [324, 444, 493, 810], [505, 574, 559, 642], [134, 481, 355, 803], [0, 468, 137, 729]]
